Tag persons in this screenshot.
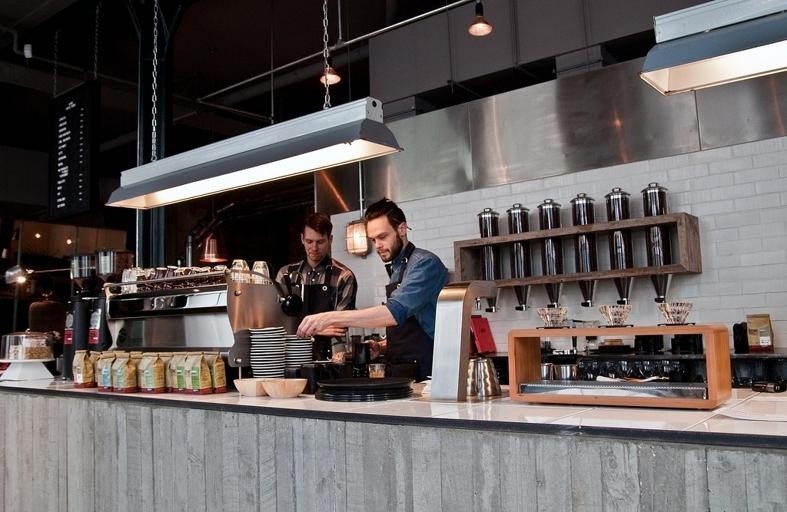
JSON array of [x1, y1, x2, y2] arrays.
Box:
[[295, 196, 450, 383], [275, 211, 357, 360]]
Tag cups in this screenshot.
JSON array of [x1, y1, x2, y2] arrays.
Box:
[[121, 264, 229, 294], [352, 342, 372, 368], [230, 259, 271, 285], [368, 363, 385, 379], [577, 360, 681, 381], [295, 364, 346, 394], [540, 363, 554, 380]]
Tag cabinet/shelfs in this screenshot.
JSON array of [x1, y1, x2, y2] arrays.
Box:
[[506, 323, 731, 410], [454, 214, 698, 289]]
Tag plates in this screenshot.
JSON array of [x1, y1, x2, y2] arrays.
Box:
[[248, 325, 313, 380]]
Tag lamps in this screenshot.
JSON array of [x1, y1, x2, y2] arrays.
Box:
[[319, 51, 342, 87], [638, 0, 787, 96], [104, 96, 406, 211], [346, 219, 371, 258], [467, 0, 493, 37]]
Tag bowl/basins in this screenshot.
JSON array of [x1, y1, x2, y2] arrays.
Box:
[[536, 307, 568, 326], [598, 304, 633, 325], [552, 364, 576, 379], [233, 376, 307, 398], [655, 302, 695, 324]]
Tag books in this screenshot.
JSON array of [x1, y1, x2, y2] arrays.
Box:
[[469, 316, 497, 354]]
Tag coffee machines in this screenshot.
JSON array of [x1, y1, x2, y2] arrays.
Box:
[[61, 248, 135, 381]]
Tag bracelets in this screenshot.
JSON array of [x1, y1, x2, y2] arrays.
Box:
[[376, 341, 383, 356]]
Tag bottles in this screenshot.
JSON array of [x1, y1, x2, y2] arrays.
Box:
[[466, 357, 503, 397], [751, 381, 785, 393]]
